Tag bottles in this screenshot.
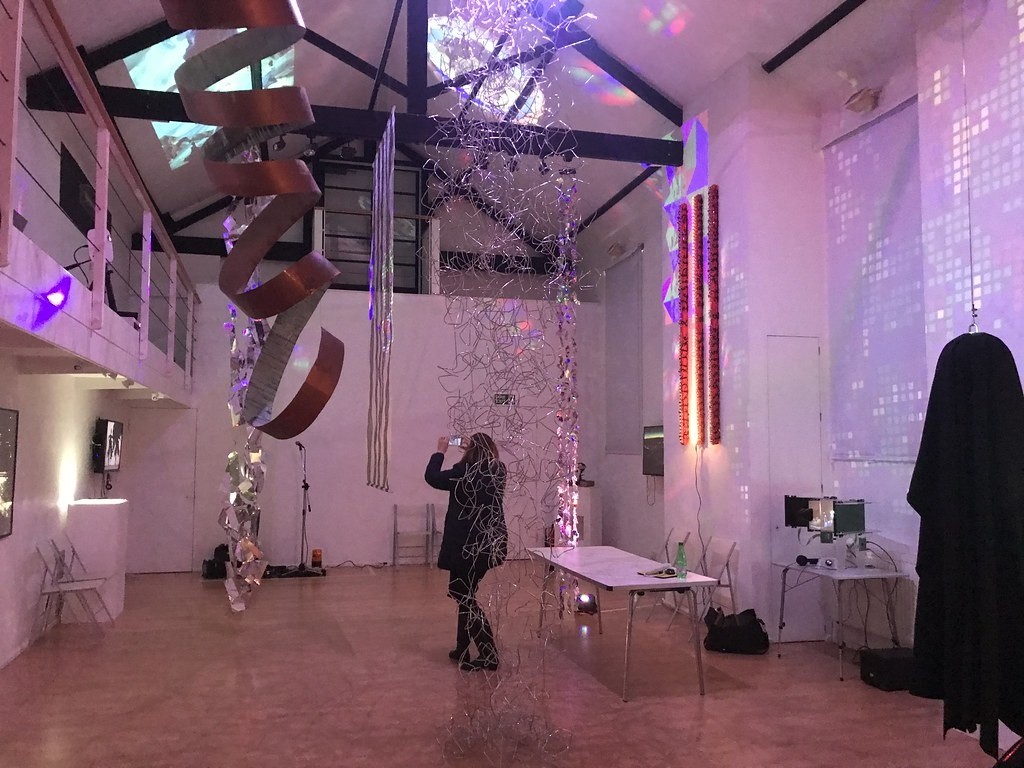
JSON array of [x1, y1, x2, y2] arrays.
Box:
[[676, 542, 686, 579]]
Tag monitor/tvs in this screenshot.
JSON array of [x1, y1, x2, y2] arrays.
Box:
[[641, 425, 665, 477], [92, 418, 124, 473]]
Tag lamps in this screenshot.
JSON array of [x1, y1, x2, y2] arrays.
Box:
[[273, 140, 286, 152], [559, 167, 576, 175], [844, 87, 877, 113], [339, 146, 358, 160], [504, 159, 517, 173], [304, 143, 317, 156], [473, 154, 488, 170], [539, 162, 549, 176], [606, 244, 624, 257]]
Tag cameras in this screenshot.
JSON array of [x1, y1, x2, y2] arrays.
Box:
[[446, 434, 462, 446]]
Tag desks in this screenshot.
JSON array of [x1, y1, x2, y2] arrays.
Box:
[[771, 562, 910, 682], [523, 546, 718, 704]]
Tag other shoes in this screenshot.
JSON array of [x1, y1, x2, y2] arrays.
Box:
[[449, 650, 470, 664], [461, 656, 498, 671]]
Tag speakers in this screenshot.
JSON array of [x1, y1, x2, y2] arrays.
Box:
[[860, 647, 919, 691]]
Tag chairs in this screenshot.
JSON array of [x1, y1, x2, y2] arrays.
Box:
[[393, 503, 433, 569], [431, 504, 449, 569], [667, 537, 738, 642], [631, 527, 696, 621], [34, 538, 105, 647], [51, 535, 115, 635]]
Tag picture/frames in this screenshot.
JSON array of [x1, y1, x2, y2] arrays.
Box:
[[0, 407, 19, 538]]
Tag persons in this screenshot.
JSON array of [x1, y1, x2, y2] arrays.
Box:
[[425, 433, 507, 672]]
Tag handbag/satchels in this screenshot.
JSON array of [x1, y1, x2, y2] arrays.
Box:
[[704, 607, 770, 654]]
